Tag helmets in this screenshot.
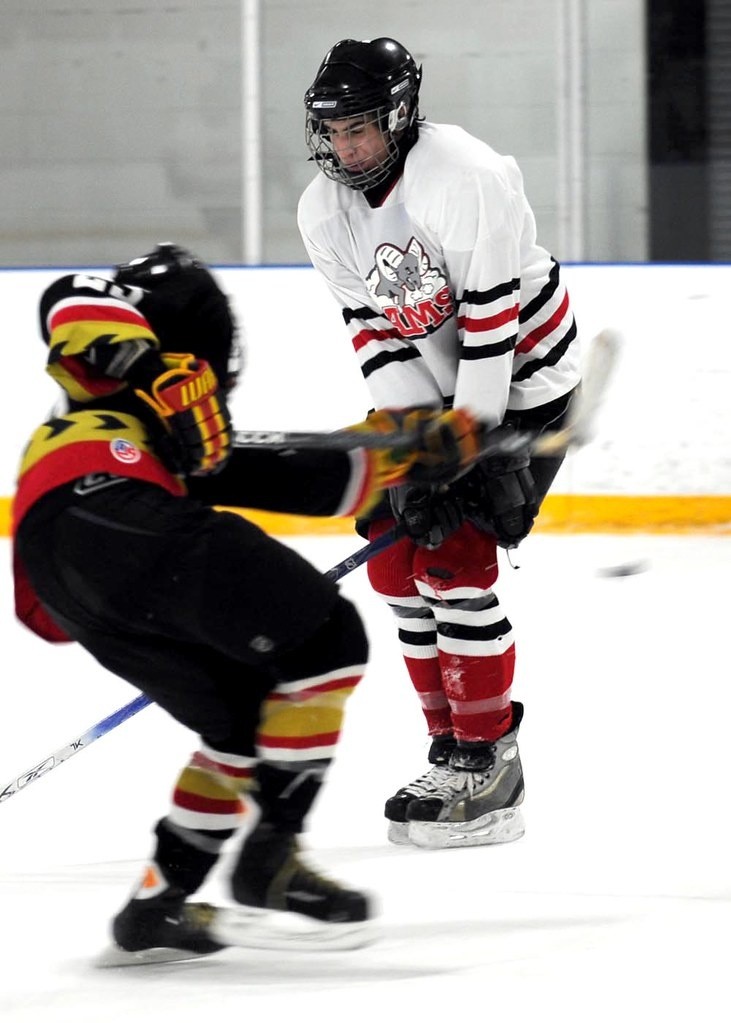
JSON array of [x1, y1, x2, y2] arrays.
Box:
[[113, 243, 231, 387], [305, 37, 424, 189]]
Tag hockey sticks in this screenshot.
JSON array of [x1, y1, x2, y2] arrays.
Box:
[[229, 326, 629, 450], [0, 518, 413, 804]]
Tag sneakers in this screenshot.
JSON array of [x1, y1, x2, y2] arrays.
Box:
[[383, 734, 462, 845], [91, 903, 231, 969], [408, 698, 526, 847], [210, 839, 380, 951]]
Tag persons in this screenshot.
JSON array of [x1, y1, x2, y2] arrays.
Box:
[[297, 37, 580, 850], [9, 243, 380, 965]]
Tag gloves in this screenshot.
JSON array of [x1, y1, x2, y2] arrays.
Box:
[[136, 352, 231, 477], [390, 468, 460, 549], [464, 448, 538, 547], [366, 408, 476, 483]]
